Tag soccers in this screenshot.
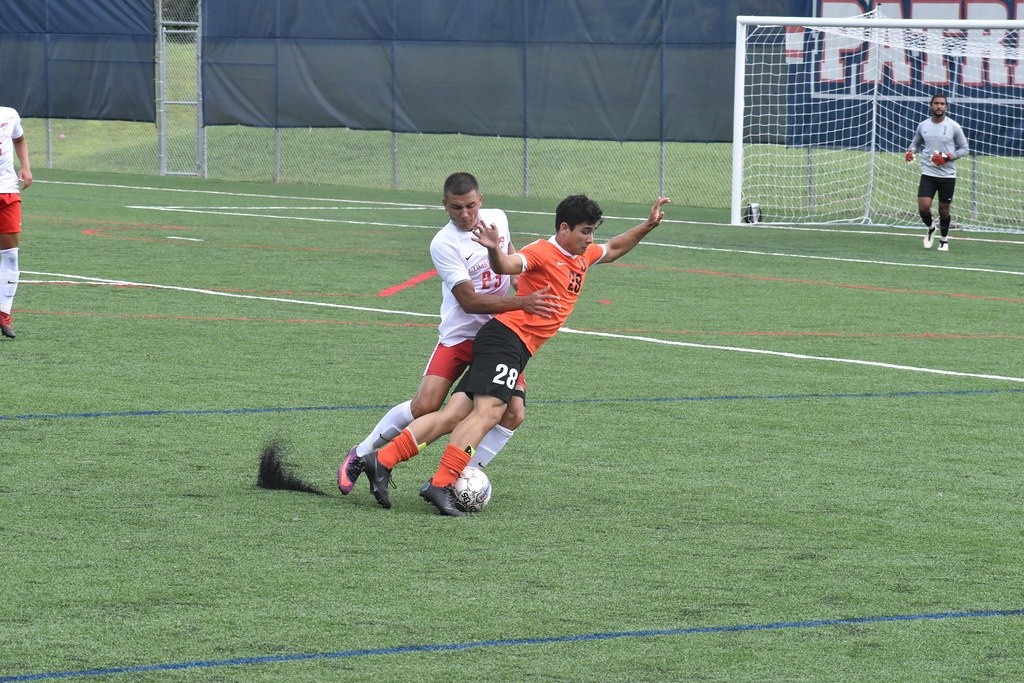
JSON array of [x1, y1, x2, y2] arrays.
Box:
[[451, 466, 492, 512]]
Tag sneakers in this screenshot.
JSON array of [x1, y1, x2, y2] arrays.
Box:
[[418, 477, 466, 518], [337, 445, 364, 496], [923, 225, 937, 249], [0, 310, 15, 339], [358, 451, 397, 509], [938, 241, 949, 251]]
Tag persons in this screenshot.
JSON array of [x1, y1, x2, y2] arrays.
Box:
[[0, 106, 32, 338], [358, 194, 671, 517], [338, 173, 562, 495], [906, 95, 969, 251]]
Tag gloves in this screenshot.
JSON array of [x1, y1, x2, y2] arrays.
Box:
[[932, 152, 952, 166], [905, 150, 913, 163]]
